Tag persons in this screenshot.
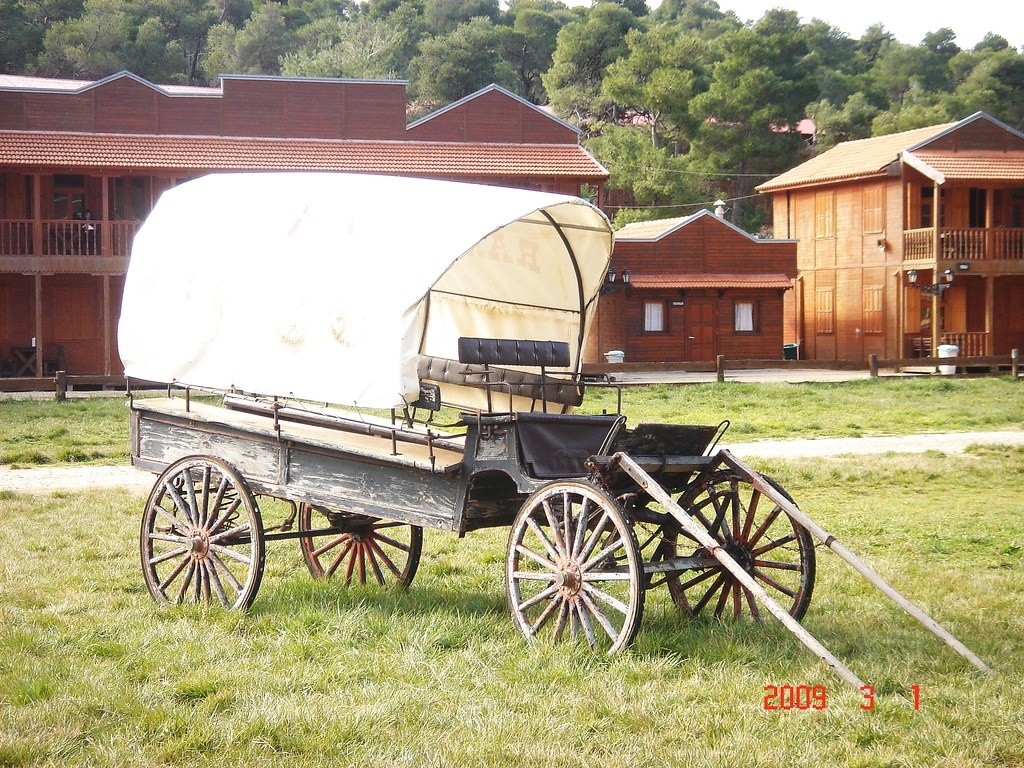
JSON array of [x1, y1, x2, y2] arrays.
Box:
[[79, 211, 97, 255]]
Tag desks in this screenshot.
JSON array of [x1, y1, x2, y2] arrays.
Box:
[[10, 345, 44, 377], [52, 228, 83, 255]]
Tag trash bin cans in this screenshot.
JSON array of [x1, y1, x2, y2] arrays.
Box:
[[937, 344, 960, 375], [603, 350, 626, 382], [783, 343, 798, 359]]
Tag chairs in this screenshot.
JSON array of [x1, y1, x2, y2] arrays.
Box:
[[25, 226, 47, 255], [44, 346, 63, 377], [0, 349, 16, 378]]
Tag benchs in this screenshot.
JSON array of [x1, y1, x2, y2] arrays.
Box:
[[457, 336, 624, 478], [125, 354, 585, 474]]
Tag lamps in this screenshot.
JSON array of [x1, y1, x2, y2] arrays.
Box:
[[907, 267, 954, 295], [600, 267, 632, 301]]
